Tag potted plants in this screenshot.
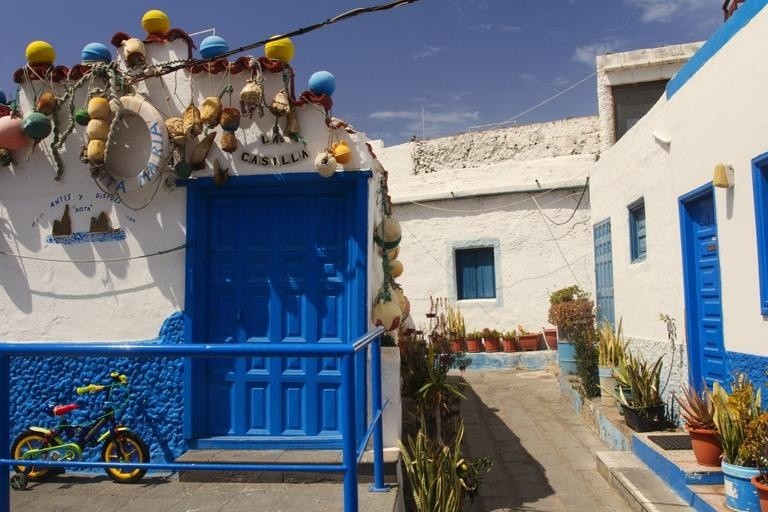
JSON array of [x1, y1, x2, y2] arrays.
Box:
[[547, 283, 595, 391], [404, 296, 542, 352], [671, 373, 768, 512], [595, 312, 683, 431]]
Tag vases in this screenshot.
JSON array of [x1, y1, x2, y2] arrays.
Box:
[[542, 329, 556, 350]]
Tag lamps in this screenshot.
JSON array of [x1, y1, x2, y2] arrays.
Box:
[[713, 163, 735, 189]]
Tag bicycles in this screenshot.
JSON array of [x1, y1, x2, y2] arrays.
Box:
[[7, 371, 149, 493]]
